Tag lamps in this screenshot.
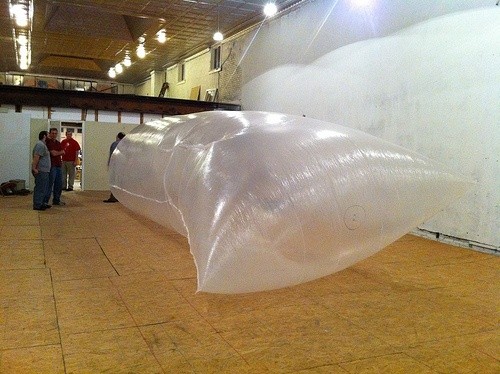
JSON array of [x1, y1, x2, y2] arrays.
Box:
[[213, 4, 223, 41]]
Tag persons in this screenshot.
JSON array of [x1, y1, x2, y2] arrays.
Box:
[[102, 132, 126, 203], [44, 127, 65, 206], [60, 131, 81, 190], [33, 131, 52, 211]]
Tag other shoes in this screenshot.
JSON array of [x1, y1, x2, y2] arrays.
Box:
[[52, 201, 65, 206], [103, 199, 116, 203], [34, 204, 51, 210], [64, 188, 73, 191]]
